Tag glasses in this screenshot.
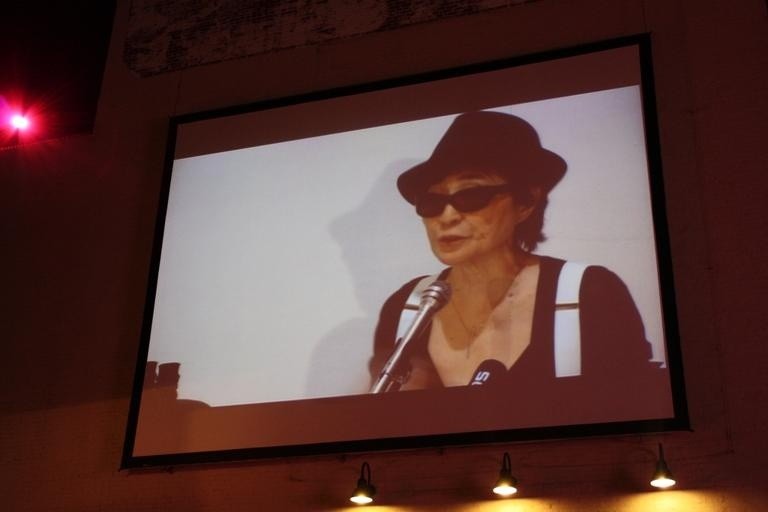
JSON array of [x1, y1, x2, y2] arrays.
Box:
[[417, 186, 507, 217]]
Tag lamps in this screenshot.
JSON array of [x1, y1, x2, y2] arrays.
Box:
[[347, 462, 377, 507], [648, 442, 677, 492], [491, 451, 519, 498]]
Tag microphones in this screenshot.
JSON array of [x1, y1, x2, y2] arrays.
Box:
[[369, 280, 452, 395]]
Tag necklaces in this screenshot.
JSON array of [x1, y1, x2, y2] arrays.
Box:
[[449, 254, 526, 359]]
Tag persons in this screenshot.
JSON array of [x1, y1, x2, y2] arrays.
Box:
[[365, 109, 655, 386]]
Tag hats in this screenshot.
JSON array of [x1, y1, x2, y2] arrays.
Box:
[[397, 111, 567, 206]]
